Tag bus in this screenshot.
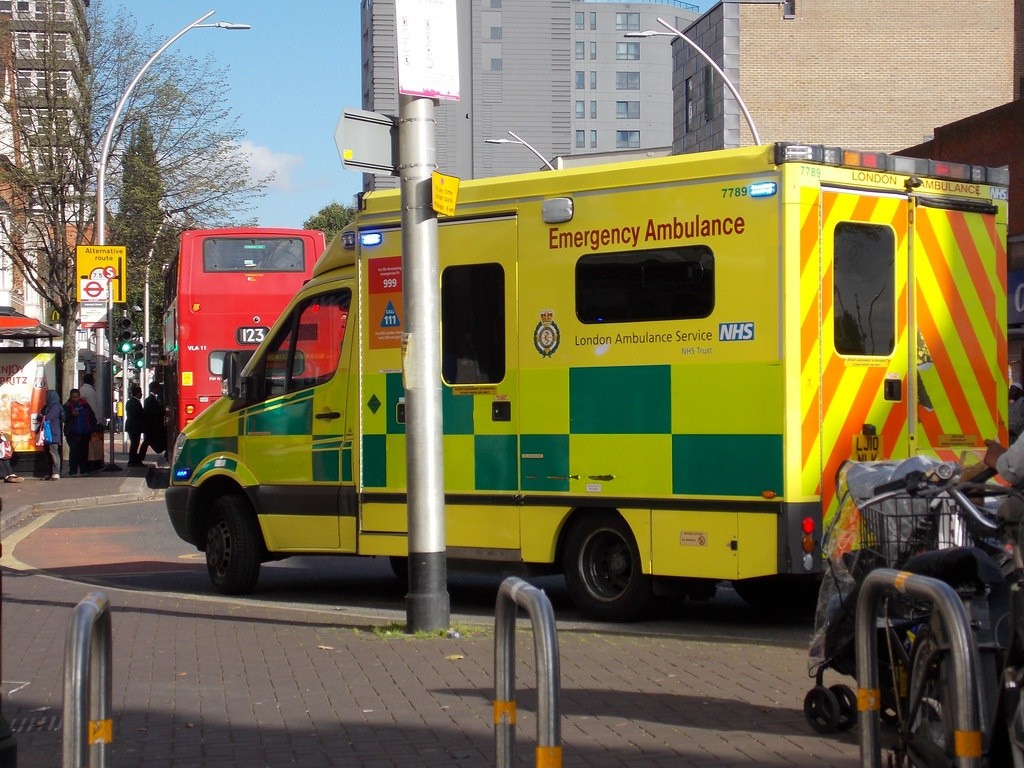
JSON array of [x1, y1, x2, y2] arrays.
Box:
[[163, 226, 326, 464]]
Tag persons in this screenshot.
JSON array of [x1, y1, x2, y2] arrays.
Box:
[[63, 374, 107, 477], [984, 431, 1024, 589], [36, 389, 65, 481], [125, 382, 166, 466]]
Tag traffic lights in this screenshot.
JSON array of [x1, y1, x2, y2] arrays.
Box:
[[135, 344, 144, 370], [117, 317, 132, 354], [146, 341, 160, 368]]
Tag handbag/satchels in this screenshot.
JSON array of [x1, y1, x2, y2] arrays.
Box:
[[35, 415, 52, 446], [0, 435, 12, 459]]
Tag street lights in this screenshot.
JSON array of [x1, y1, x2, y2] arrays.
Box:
[[142, 209, 215, 450], [624, 29, 761, 146], [95, 22, 252, 473], [484, 140, 555, 171]]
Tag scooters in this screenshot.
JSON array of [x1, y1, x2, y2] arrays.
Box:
[[802, 466, 1023, 768]]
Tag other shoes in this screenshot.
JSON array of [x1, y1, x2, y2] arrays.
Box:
[[127, 462, 147, 467], [69, 473, 87, 477], [41, 474, 60, 481], [4, 476, 24, 483]]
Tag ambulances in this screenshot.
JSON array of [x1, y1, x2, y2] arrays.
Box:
[[166, 140, 1012, 622]]
